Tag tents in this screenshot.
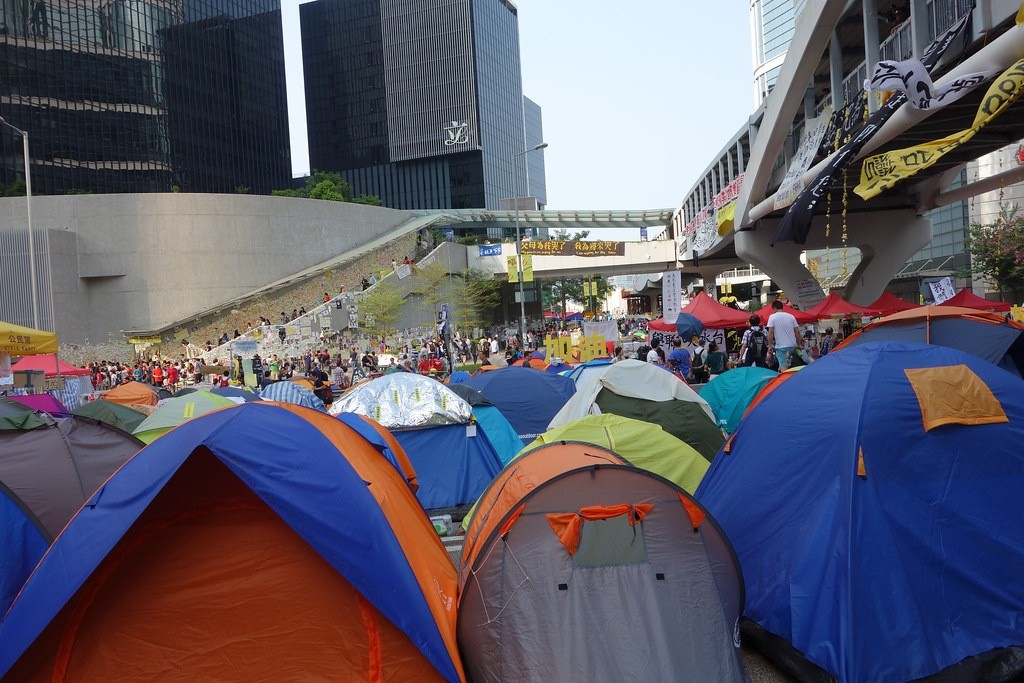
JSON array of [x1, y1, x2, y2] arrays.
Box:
[[648, 289, 757, 331], [865, 287, 929, 320], [933, 288, 1013, 315], [804, 291, 882, 320], [0, 321, 62, 403], [751, 296, 820, 331], [0, 305, 1024, 683], [10, 352, 91, 403]]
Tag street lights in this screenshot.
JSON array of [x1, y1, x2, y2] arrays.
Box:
[[510, 142, 548, 350], [0, 116, 38, 330]]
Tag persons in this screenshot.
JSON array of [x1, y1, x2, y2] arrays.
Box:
[[73, 300, 860, 389], [473, 236, 534, 244], [392, 260, 397, 271], [368, 273, 377, 284], [324, 292, 330, 302], [339, 284, 346, 294], [403, 256, 415, 265], [361, 278, 369, 290]]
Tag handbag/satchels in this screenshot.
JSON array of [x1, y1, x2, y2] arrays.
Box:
[[748, 328, 768, 357], [692, 349, 705, 368]]
